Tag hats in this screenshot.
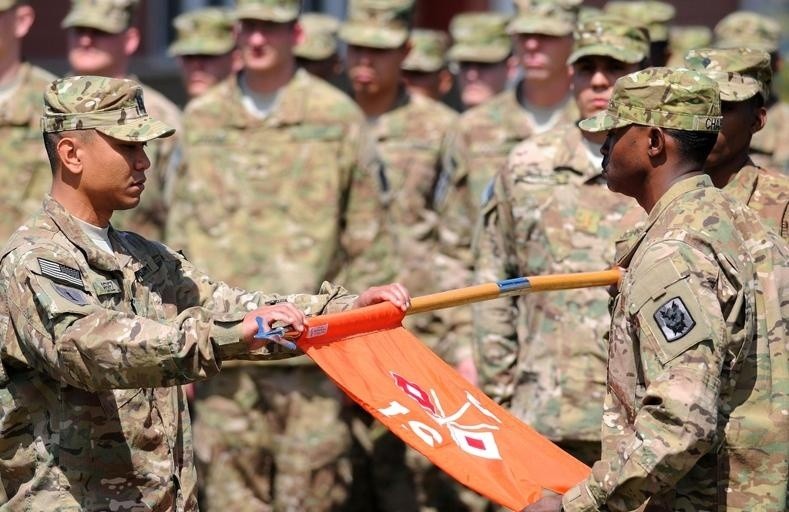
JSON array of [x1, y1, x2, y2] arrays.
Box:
[[502, 0, 713, 66], [38, 74, 177, 144], [398, 28, 448, 71], [578, 67, 724, 133], [229, 0, 301, 23], [338, 0, 413, 49], [685, 44, 773, 105], [443, 12, 514, 63], [714, 11, 782, 54], [290, 14, 340, 60], [62, 1, 131, 35], [167, 7, 239, 57]]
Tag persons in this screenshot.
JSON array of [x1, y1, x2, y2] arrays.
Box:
[[0, 2, 184, 249], [167, 0, 650, 510], [591, 11, 789, 242], [0, 75, 412, 512], [520, 69, 789, 512]]
